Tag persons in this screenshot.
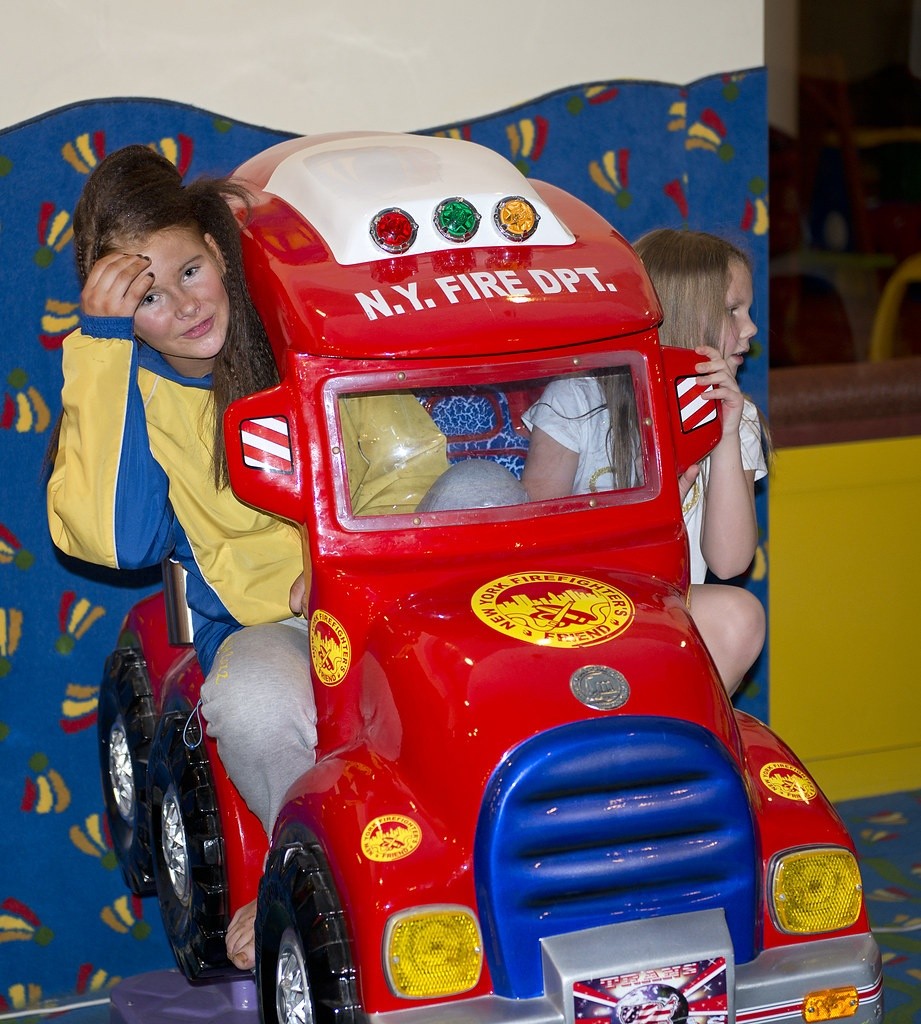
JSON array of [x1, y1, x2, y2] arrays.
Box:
[[45, 145, 530, 971], [523, 228, 772, 703]]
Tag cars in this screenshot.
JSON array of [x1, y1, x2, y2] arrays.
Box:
[[95, 132, 886, 1024]]
